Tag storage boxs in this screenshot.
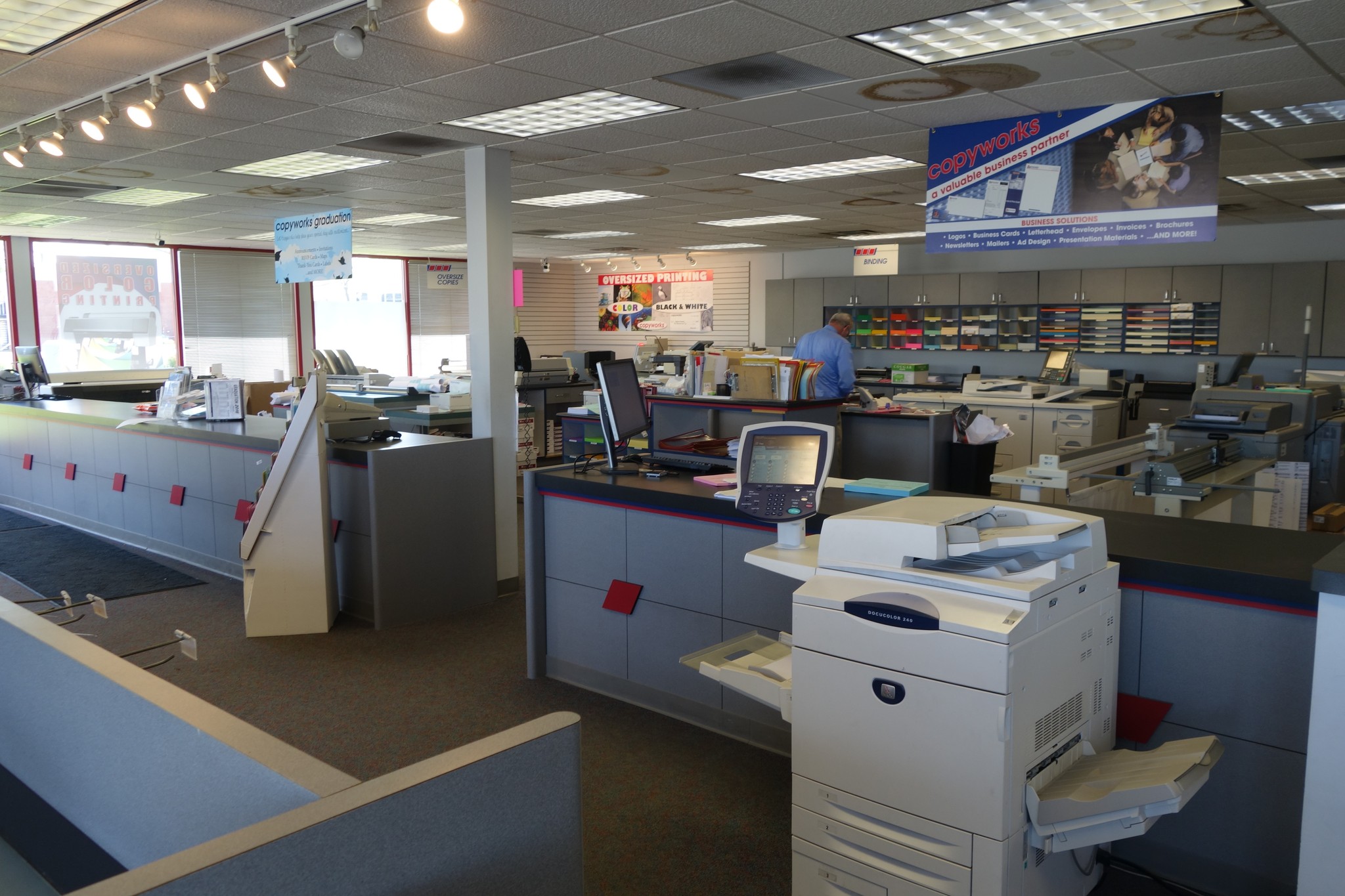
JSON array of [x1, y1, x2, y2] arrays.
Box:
[[517, 418, 563, 477], [429, 393, 471, 411]]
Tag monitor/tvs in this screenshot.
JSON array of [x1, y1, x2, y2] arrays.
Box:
[[595, 358, 650, 474], [14, 346, 51, 400], [1038, 347, 1076, 382], [740, 425, 828, 489], [689, 339, 714, 351]]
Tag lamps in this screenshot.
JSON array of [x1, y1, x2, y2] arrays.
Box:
[[606, 259, 617, 271], [685, 252, 697, 266], [4, 124, 37, 168], [39, 110, 74, 156], [334, 0, 383, 59], [579, 260, 591, 273], [81, 93, 119, 140], [656, 254, 666, 269], [127, 75, 166, 128], [262, 25, 312, 87], [184, 54, 230, 109], [630, 257, 641, 270], [428, 0, 464, 35]]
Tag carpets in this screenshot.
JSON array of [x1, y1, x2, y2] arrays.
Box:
[[0, 508, 48, 531], [0, 525, 208, 607]]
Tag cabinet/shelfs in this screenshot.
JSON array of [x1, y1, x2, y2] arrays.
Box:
[[893, 388, 1345, 536], [557, 412, 647, 464]]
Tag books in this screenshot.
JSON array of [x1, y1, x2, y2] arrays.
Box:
[[156, 366, 247, 420], [687, 347, 825, 402]]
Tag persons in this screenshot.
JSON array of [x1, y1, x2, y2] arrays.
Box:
[[792, 313, 857, 479]]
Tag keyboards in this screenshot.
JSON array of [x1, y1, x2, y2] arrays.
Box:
[[37, 394, 72, 401]]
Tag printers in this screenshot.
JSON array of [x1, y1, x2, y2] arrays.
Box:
[[514, 356, 576, 386], [679, 496, 1226, 896]]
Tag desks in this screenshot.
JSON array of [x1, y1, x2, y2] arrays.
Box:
[[646, 394, 862, 474], [522, 453, 1320, 896], [515, 458, 1163, 554], [517, 382, 594, 458], [841, 409, 983, 490], [385, 407, 536, 435]]
